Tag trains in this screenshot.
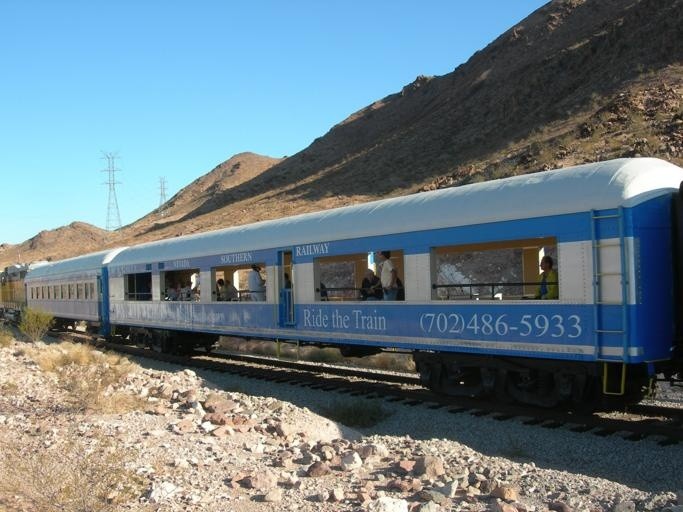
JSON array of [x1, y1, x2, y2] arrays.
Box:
[[1, 157, 683, 409]]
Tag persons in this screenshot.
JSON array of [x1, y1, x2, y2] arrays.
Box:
[[377, 251, 398, 301], [360, 268, 383, 301], [520, 256, 558, 299], [395, 275, 405, 301], [247, 264, 266, 302], [284, 272, 292, 290], [140, 278, 239, 302]]
